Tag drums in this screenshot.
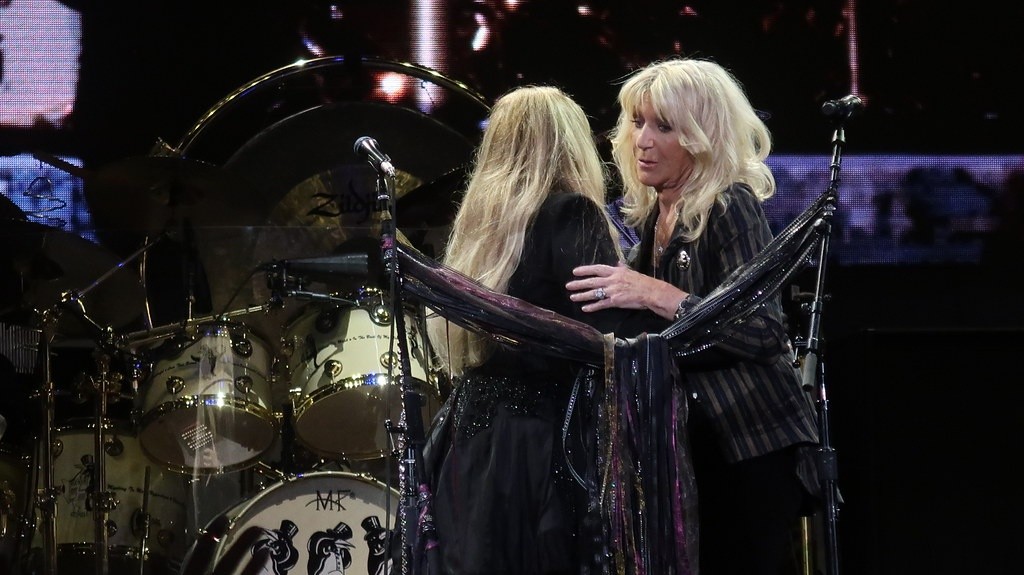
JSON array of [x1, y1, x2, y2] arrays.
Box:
[[175, 466, 401, 575], [278, 288, 447, 462], [132, 316, 287, 478], [20, 411, 193, 574]]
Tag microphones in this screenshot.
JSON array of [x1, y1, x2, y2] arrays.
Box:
[[354, 136, 395, 179], [822, 94, 861, 116]]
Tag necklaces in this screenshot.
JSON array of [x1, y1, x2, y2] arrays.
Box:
[[654, 213, 678, 256]]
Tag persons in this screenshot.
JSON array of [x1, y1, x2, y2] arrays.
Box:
[[423, 85, 622, 539], [565, 58, 820, 539]]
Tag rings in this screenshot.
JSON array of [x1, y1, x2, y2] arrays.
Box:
[[594, 287, 606, 299]]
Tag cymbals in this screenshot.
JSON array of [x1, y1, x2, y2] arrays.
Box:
[[0, 216, 149, 338], [81, 152, 244, 220]]
